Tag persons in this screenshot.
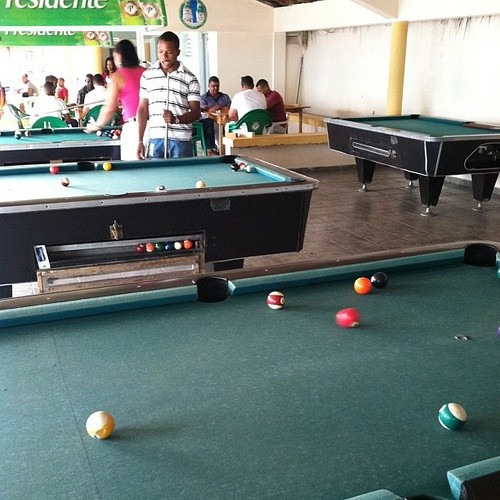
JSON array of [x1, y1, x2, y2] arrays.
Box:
[[0, 57, 124, 130], [83, 39, 147, 161], [224, 75, 268, 135], [199, 75, 232, 156], [136, 31, 201, 160], [256, 79, 287, 134]]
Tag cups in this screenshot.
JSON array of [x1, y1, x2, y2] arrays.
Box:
[[44, 122, 51, 129]]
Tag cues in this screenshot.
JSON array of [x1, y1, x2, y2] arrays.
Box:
[[164, 72, 170, 159]]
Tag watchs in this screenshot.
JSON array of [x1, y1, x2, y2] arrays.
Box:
[[174, 115, 180, 124]]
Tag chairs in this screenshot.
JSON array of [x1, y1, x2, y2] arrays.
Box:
[[191, 123, 208, 156], [229, 108, 271, 153], [266, 112, 292, 135]]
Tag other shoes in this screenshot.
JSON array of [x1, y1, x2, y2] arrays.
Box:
[[208, 150, 213, 156]]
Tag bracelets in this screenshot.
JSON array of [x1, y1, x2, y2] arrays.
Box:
[[138, 140, 144, 144]]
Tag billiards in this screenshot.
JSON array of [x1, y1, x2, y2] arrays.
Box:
[[266, 291, 285, 310], [438, 403, 468, 431], [85, 410, 115, 440], [353, 276, 372, 295], [96, 130, 103, 137], [49, 166, 59, 174], [196, 180, 206, 188], [370, 272, 388, 289], [60, 177, 69, 187], [15, 131, 21, 136], [15, 134, 21, 140], [103, 162, 112, 171], [25, 131, 31, 137], [230, 162, 252, 173], [136, 239, 193, 253], [156, 185, 168, 191], [335, 308, 360, 329], [105, 129, 121, 140]]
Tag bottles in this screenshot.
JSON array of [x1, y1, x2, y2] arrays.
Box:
[[97, 31, 112, 46], [118, 0, 146, 26], [82, 31, 99, 46], [138, 0, 164, 25]]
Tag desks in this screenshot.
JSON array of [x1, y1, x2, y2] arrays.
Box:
[[323, 113, 500, 216], [208, 111, 237, 155], [0, 125, 122, 166], [1, 242, 500, 500], [0, 152, 320, 289]]
